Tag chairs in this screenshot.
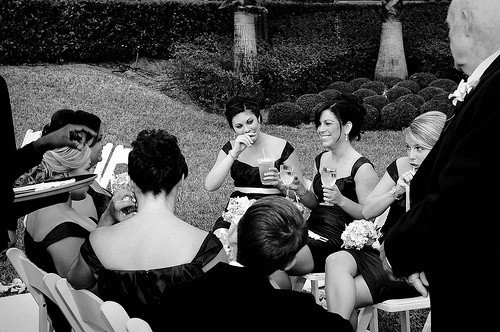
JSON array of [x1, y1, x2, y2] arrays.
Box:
[[7, 247, 152, 332], [289, 273, 433, 332], [20, 129, 133, 228]]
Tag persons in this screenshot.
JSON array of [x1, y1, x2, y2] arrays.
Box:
[[324, 110, 447, 332], [203, 96, 306, 260], [379, 0, 500, 332], [0, 74, 99, 257], [67, 128, 231, 332], [42, 108, 114, 224], [269, 93, 379, 290], [167, 194, 357, 332], [14, 138, 102, 332]]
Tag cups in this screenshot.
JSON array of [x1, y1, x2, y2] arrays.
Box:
[[259, 164, 274, 183]]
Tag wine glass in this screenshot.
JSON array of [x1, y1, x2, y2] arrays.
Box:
[[280, 165, 294, 202], [319, 167, 336, 206]]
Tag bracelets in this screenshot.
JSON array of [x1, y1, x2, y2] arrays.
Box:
[[297, 188, 308, 197], [229, 150, 238, 160], [387, 186, 403, 200]]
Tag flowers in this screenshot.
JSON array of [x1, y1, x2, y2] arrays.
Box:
[[449, 79, 478, 106], [341, 219, 384, 251]]
